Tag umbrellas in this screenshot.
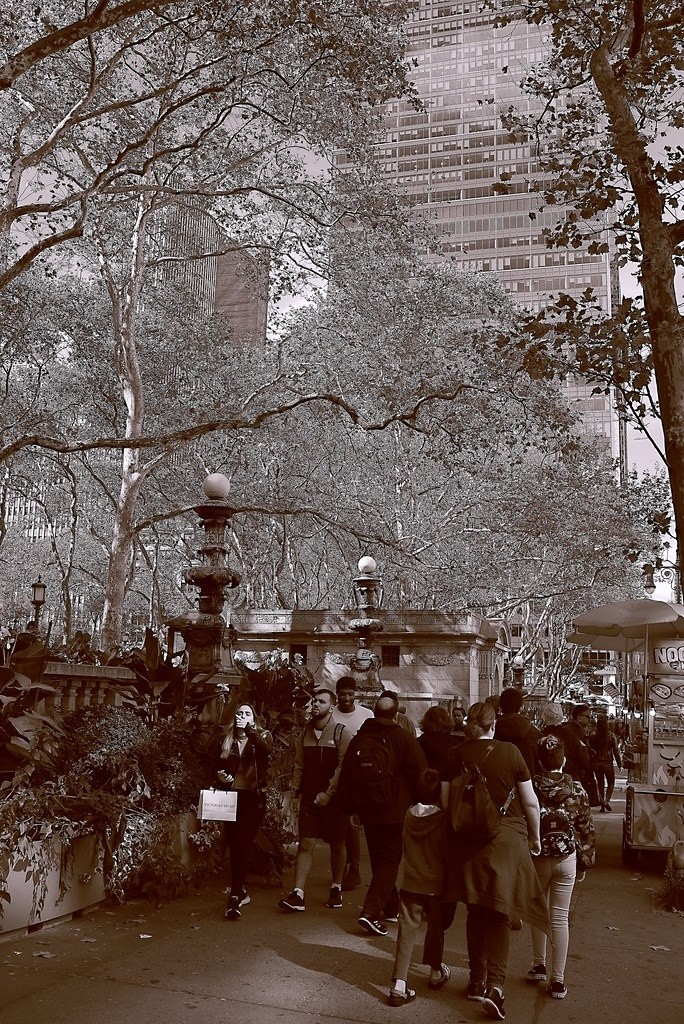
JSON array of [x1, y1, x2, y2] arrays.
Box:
[[567, 598, 684, 722]]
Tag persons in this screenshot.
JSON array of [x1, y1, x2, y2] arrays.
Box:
[[213, 702, 274, 918], [279, 676, 627, 1020]]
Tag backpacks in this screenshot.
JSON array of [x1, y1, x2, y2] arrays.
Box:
[[539, 805, 576, 856], [446, 739, 516, 843], [348, 728, 400, 808]]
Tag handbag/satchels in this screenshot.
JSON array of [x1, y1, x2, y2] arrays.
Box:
[[196, 777, 238, 822]]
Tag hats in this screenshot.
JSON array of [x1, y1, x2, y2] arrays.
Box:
[[336, 676, 358, 691]]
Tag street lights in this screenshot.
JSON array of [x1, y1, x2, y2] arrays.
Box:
[[29, 573, 47, 641]]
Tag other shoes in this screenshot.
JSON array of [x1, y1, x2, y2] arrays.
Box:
[[600, 806, 605, 812], [604, 801, 611, 811]]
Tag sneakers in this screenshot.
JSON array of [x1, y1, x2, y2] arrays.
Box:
[[526, 963, 547, 981], [278, 891, 305, 911], [340, 864, 362, 891], [546, 980, 567, 999], [467, 980, 487, 1002], [238, 889, 251, 907], [326, 887, 343, 908], [357, 911, 389, 936], [225, 896, 241, 919], [482, 986, 506, 1020], [385, 912, 400, 923]]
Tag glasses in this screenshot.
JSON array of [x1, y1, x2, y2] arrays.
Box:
[[580, 715, 591, 720]]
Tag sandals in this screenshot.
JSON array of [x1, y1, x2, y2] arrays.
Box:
[[428, 962, 451, 990], [388, 988, 416, 1006]]
